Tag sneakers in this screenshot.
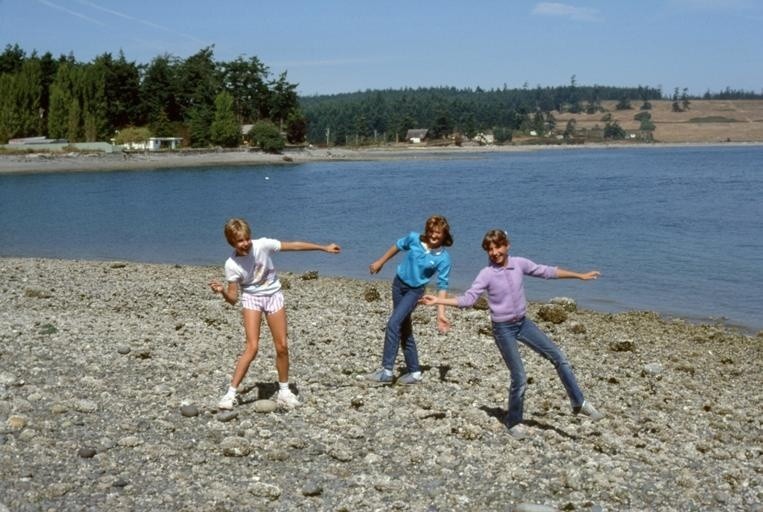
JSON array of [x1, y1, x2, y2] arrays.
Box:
[[512, 423, 527, 440], [278, 390, 299, 408], [365, 369, 393, 384], [580, 402, 603, 420], [218, 393, 236, 409], [397, 371, 422, 385]]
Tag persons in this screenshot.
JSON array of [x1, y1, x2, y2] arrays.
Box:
[[364, 215, 453, 385], [207, 218, 342, 410], [418, 229, 600, 439]]
